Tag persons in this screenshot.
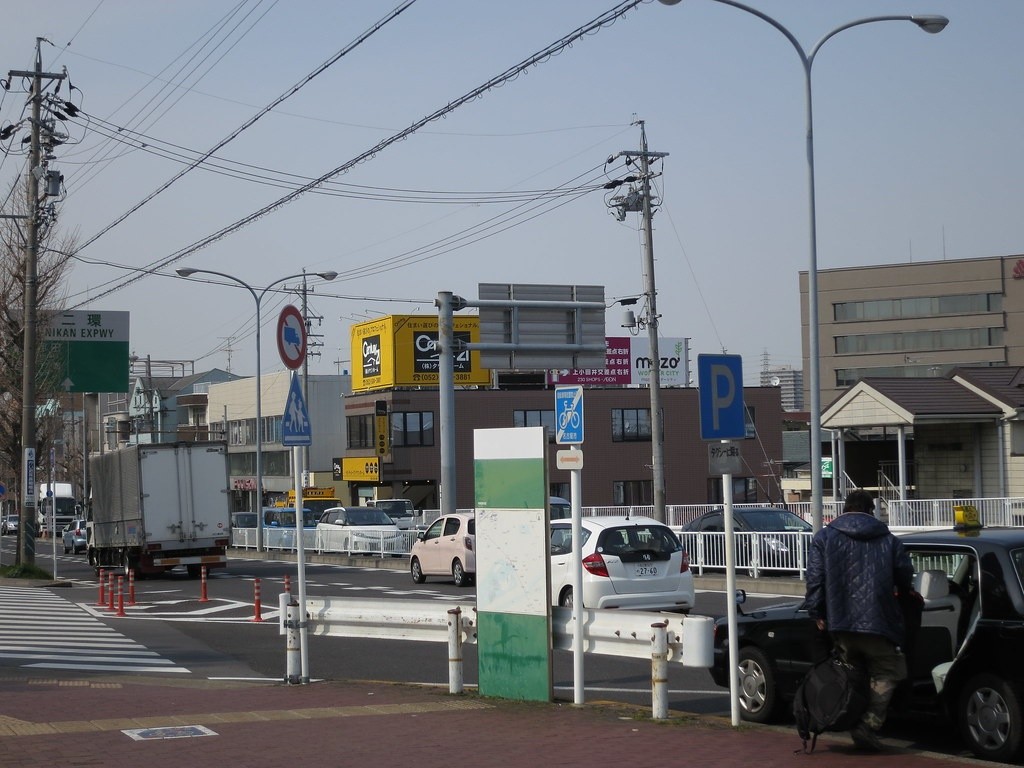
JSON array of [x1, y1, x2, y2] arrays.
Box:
[[805, 488, 915, 752]]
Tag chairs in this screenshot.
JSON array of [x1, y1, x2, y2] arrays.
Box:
[[287, 516, 292, 524], [612, 531, 626, 546], [562, 538, 571, 549], [916, 568, 963, 659]]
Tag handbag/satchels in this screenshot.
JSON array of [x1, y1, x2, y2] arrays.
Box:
[[793, 657, 858, 755]]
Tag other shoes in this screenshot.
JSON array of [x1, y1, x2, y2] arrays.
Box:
[[852, 725, 885, 753]]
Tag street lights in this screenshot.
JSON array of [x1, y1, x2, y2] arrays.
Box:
[[175, 267, 338, 553], [658, 0, 949, 540]]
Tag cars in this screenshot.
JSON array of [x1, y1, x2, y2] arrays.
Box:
[[262, 508, 318, 552], [708, 526, 1024, 766], [551, 516, 694, 614], [410, 513, 476, 587], [315, 506, 404, 558], [231, 512, 257, 549], [62, 519, 87, 555], [678, 507, 812, 576], [3, 515, 19, 535]]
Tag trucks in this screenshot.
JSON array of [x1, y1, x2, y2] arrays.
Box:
[[366, 499, 423, 545], [272, 487, 343, 527], [37, 483, 78, 537], [75, 440, 233, 580]]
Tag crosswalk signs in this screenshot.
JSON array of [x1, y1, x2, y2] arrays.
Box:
[[282, 371, 312, 446]]
